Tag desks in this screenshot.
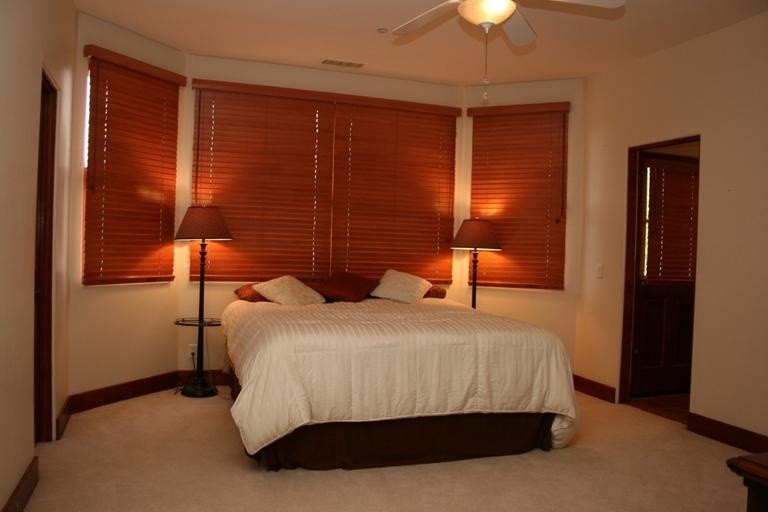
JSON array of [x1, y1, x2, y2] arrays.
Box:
[[171, 317, 223, 398]]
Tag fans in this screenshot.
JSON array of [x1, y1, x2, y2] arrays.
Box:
[[388, 0, 627, 47]]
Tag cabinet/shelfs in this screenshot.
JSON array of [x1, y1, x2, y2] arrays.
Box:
[[726, 452, 768, 512]]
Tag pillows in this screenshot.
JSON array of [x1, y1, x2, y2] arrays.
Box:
[[233, 268, 447, 306]]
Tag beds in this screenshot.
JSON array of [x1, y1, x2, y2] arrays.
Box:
[[225, 296, 577, 470]]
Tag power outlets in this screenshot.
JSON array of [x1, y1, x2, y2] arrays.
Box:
[[187, 343, 198, 358]]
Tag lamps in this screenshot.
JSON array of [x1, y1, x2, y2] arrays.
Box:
[[456, 0, 519, 36], [449, 215, 503, 310], [171, 203, 234, 398]]
